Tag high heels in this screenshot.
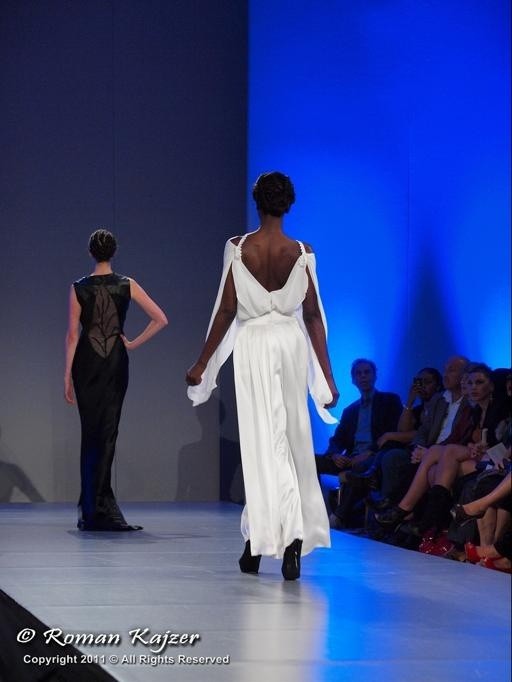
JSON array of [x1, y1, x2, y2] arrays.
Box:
[[327, 496, 512, 573], [238, 538, 261, 574], [280, 535, 306, 581]]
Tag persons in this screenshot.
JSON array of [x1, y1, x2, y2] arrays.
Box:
[[314, 352, 512, 575], [183, 169, 341, 581], [63, 227, 170, 532]]
